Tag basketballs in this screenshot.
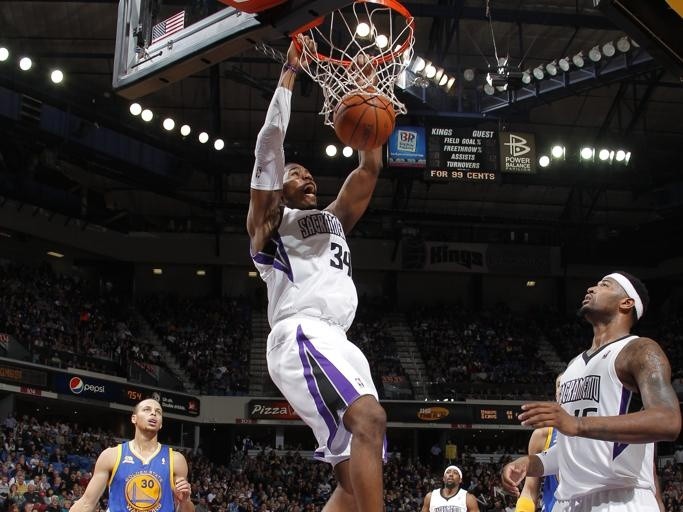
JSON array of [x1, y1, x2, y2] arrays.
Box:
[[333, 88, 395, 151]]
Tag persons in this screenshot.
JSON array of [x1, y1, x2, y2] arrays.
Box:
[[0, 256, 247, 398], [347, 293, 417, 400], [70, 398, 196, 512], [246, 33, 387, 512], [399, 292, 681, 401], [501, 271, 682, 512], [1, 412, 682, 511], [515, 372, 565, 512]]
